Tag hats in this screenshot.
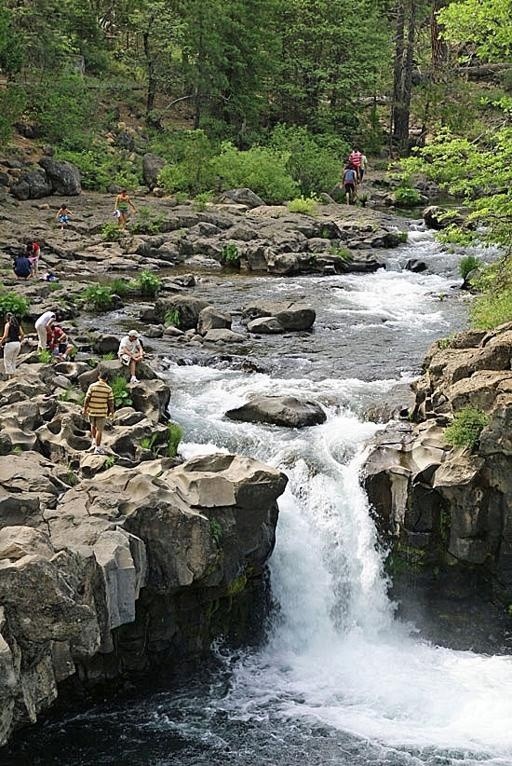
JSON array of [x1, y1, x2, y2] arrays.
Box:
[[127, 329, 141, 338]]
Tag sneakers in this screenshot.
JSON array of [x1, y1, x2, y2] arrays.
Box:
[[92, 442, 105, 454], [130, 379, 140, 384], [122, 354, 128, 363]]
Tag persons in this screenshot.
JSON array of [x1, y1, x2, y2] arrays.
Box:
[[360, 149, 368, 180], [13, 252, 32, 281], [1, 312, 25, 381], [350, 147, 362, 177], [50, 324, 68, 345], [35, 310, 60, 353], [27, 240, 41, 277], [48, 333, 74, 361], [82, 371, 114, 455], [342, 164, 358, 205], [113, 189, 136, 227], [117, 329, 144, 382], [56, 203, 73, 229]]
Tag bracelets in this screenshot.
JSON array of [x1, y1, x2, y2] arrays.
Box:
[[52, 335, 55, 338]]
